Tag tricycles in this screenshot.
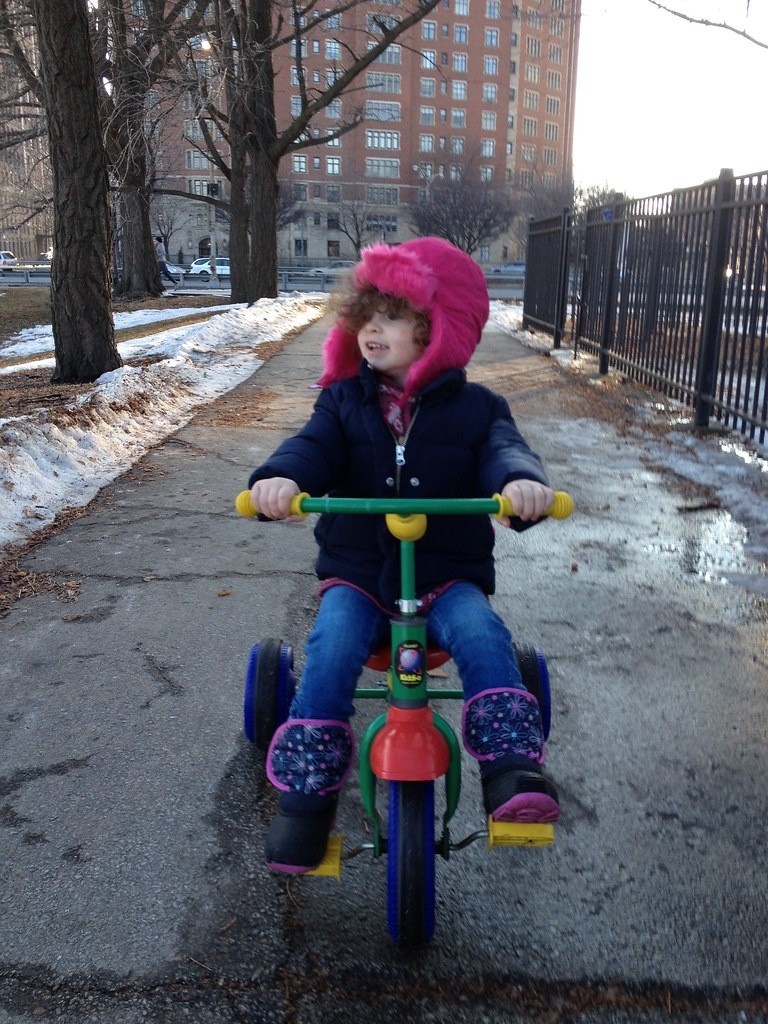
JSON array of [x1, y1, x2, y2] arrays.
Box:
[[237, 488, 578, 971]]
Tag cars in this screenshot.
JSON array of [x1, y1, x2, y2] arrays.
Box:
[[306, 260, 361, 279], [161, 260, 187, 283], [491, 261, 525, 273], [189, 256, 233, 282], [0, 251, 19, 272]]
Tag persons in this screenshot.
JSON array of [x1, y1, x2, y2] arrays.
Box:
[[154, 237, 177, 284], [248, 236, 561, 876]]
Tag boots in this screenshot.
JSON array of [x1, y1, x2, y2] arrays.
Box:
[[266, 717, 357, 872], [462, 687, 561, 821]]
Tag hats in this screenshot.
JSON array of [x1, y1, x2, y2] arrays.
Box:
[[307, 238, 491, 407]]
